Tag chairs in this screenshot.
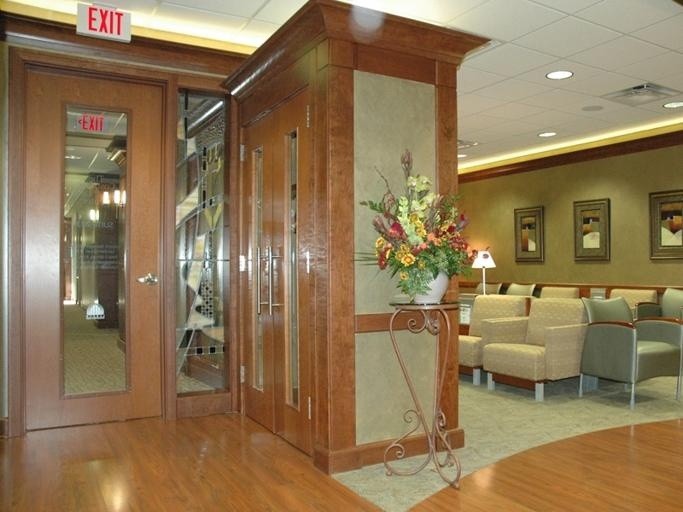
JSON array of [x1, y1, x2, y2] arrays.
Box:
[[459, 294, 532, 386], [635, 288, 683, 320], [474, 282, 503, 295], [484, 295, 589, 402], [581, 297, 682, 404], [504, 283, 536, 295]]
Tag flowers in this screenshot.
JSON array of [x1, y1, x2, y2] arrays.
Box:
[[354, 149, 488, 303]]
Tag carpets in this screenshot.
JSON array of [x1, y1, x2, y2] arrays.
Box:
[[329, 373, 683, 512]]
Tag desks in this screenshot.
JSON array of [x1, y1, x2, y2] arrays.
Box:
[[383, 302, 462, 489]]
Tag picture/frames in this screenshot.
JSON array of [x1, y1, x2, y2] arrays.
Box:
[[514, 205, 545, 262], [649, 189, 683, 259], [573, 198, 611, 261]]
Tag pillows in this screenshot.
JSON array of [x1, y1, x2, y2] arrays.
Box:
[[609, 289, 657, 318], [539, 286, 579, 298], [468, 294, 530, 337], [525, 298, 586, 346]]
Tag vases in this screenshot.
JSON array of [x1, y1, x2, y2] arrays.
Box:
[[410, 265, 450, 305]]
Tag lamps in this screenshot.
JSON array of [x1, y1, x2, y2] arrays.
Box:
[[471, 250, 497, 294], [99, 188, 126, 205]]
